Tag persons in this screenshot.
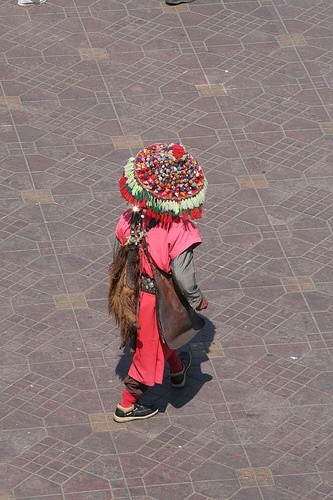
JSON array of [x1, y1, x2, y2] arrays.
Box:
[[106, 142, 209, 423]]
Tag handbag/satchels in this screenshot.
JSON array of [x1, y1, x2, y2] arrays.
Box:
[[151, 264, 206, 350]]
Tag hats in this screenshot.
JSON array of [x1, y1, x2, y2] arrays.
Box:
[[119, 142, 208, 222]]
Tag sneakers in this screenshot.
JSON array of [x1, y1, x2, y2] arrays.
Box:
[[169, 351, 192, 388], [114, 401, 160, 422]]
